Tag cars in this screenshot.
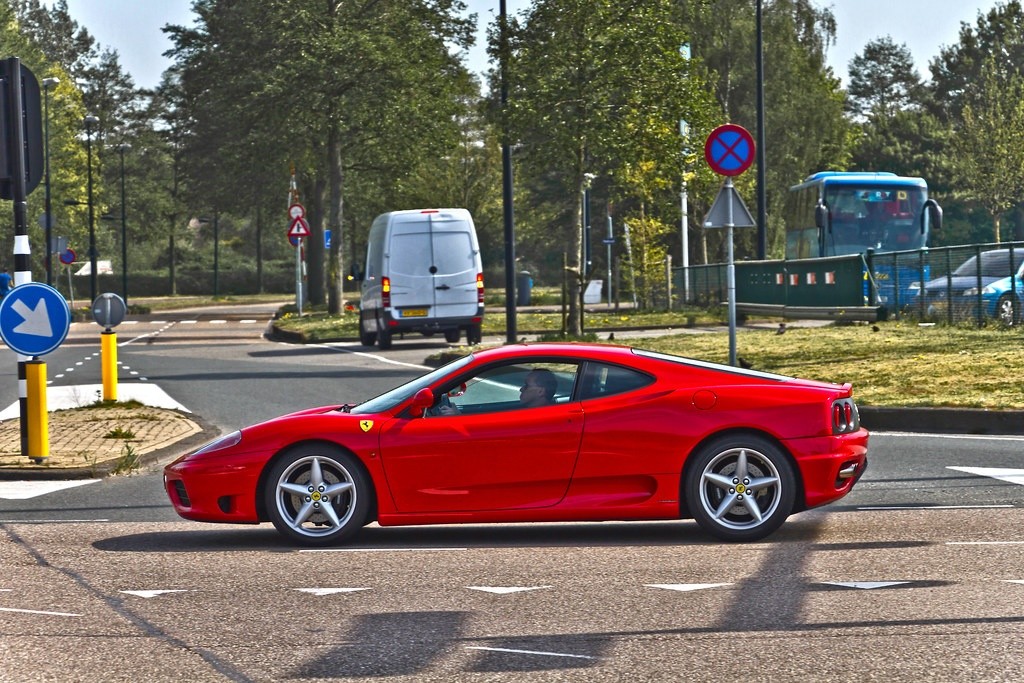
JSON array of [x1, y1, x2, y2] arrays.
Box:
[[916, 249, 1024, 329], [162, 342, 871, 549]]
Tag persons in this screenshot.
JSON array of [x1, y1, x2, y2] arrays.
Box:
[[0, 267, 14, 296], [440, 368, 558, 417]]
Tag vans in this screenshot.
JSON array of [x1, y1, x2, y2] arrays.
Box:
[[354, 208, 485, 350]]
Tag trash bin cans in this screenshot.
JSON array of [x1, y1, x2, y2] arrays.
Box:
[[517, 271, 532, 306]]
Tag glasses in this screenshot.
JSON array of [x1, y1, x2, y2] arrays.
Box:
[[524, 384, 544, 390]]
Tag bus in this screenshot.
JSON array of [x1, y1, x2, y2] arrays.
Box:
[[784, 171, 944, 309]]
